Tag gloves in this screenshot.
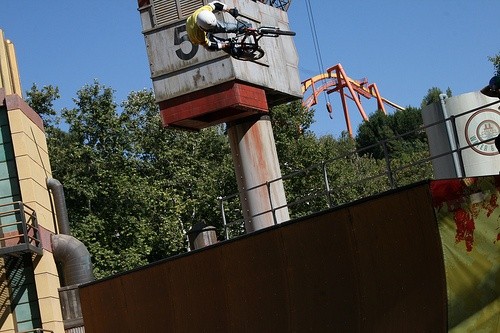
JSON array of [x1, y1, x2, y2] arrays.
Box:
[[229, 9, 238, 17], [224, 42, 235, 49]]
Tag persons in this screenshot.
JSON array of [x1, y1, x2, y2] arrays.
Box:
[[185, 0, 256, 57]]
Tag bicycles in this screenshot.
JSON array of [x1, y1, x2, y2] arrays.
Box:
[[224, 6, 296, 61]]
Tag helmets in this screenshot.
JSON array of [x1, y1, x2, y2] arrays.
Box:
[[196, 10, 218, 33]]
